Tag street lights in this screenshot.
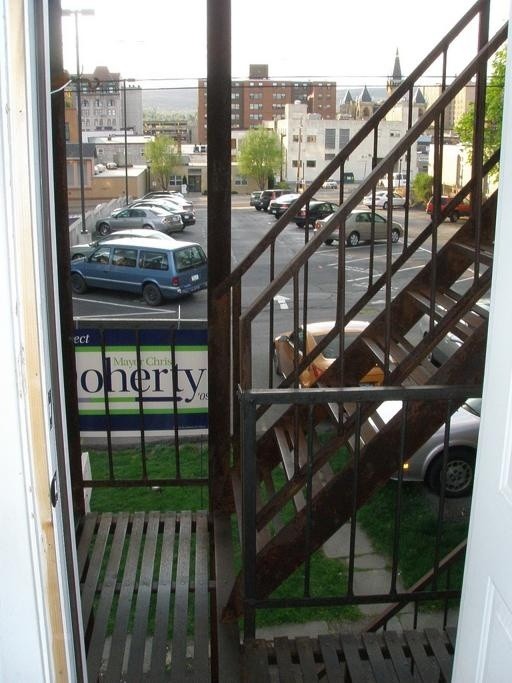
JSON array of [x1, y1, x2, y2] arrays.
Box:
[[295, 123, 304, 192], [459, 148, 464, 186], [397, 156, 402, 188], [61, 9, 98, 233]]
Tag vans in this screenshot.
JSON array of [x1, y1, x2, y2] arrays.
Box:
[[69, 237, 209, 307]]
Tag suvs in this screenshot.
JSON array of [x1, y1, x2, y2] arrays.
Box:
[[250, 191, 264, 210], [426, 195, 472, 223], [379, 173, 407, 189], [260, 190, 296, 211]]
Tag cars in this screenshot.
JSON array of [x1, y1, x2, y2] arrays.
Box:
[[271, 320, 392, 389], [422, 299, 491, 367], [350, 397, 482, 498], [95, 190, 195, 235], [314, 210, 403, 247], [323, 178, 337, 189], [71, 228, 179, 262], [364, 191, 412, 209], [267, 193, 318, 219], [291, 201, 340, 229]]
